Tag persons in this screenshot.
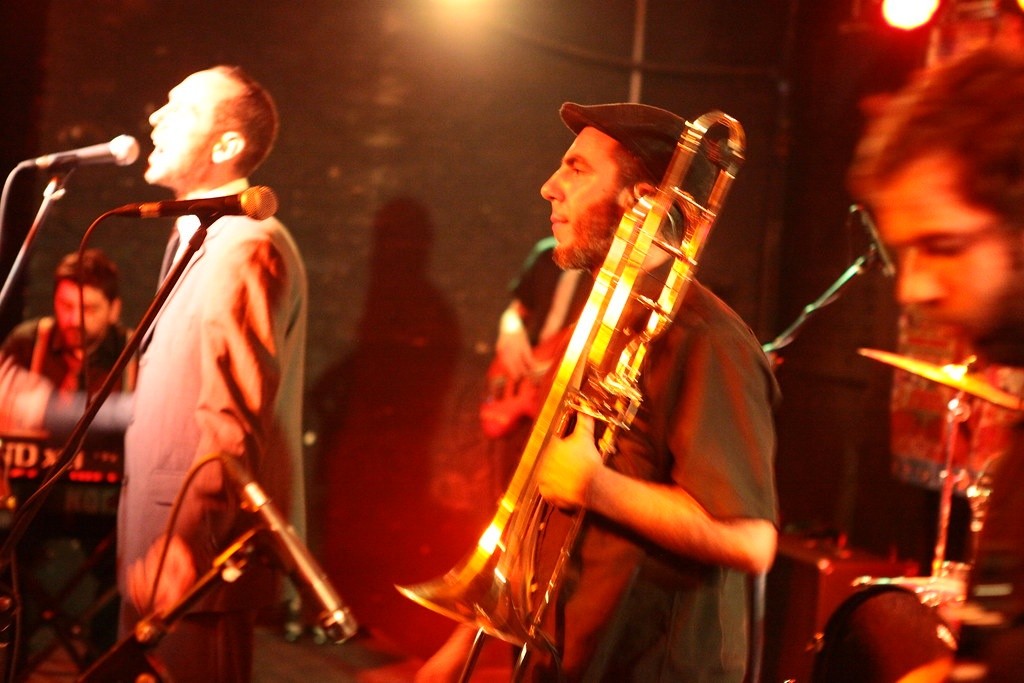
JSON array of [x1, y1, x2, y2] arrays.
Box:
[[412, 101, 786, 683], [813, 46, 1024, 683], [0, 247, 138, 666], [493, 235, 598, 490], [114, 65, 307, 683]]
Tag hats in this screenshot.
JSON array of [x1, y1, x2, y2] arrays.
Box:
[[559, 103, 717, 208]]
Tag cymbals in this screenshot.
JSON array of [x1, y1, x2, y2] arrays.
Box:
[[855, 345, 1023, 415]]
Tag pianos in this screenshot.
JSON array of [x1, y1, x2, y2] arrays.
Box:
[[1, 434, 125, 683]]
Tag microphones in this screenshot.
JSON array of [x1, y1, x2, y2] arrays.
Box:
[[25, 134, 142, 172], [849, 203, 899, 280], [220, 454, 361, 647], [109, 185, 281, 222]]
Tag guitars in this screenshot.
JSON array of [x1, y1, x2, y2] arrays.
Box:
[[479, 322, 576, 439]]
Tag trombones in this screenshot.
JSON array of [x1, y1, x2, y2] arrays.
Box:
[[390, 108, 751, 682]]
